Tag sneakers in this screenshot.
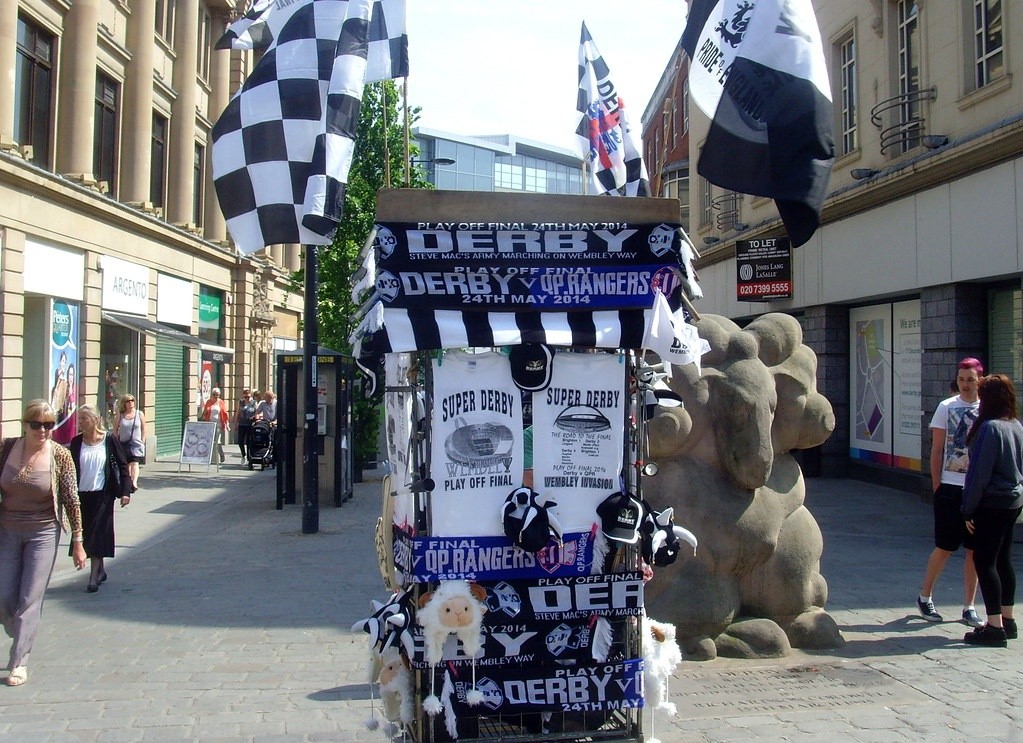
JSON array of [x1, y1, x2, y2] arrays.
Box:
[[917, 595, 942, 623], [963, 606, 985, 627]]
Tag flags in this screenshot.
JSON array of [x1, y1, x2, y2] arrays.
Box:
[[681, 0, 836, 249], [575, 19, 650, 197], [212, 0, 409, 255], [642, 286, 711, 375]]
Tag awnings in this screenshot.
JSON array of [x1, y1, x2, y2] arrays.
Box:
[[104, 312, 235, 363]]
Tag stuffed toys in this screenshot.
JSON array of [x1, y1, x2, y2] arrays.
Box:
[[631, 607, 682, 743], [364, 640, 414, 743], [418, 580, 487, 715]]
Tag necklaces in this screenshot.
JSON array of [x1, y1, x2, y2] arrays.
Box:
[[20, 437, 40, 483]]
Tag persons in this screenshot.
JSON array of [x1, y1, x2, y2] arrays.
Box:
[[915, 357, 1023, 647], [233, 390, 277, 464], [112, 394, 146, 493], [68, 404, 131, 592], [202, 388, 231, 462], [0, 398, 87, 686], [51, 351, 77, 445]]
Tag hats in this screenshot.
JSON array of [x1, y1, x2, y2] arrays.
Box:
[[597, 490, 646, 545], [508, 343, 557, 390], [643, 507, 698, 567], [500, 486, 565, 550], [641, 618, 682, 743], [351, 580, 487, 737]]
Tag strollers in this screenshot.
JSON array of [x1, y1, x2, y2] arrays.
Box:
[[246, 417, 276, 470]]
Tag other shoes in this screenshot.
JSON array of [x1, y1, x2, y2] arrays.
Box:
[[964, 624, 1006, 647], [221, 454, 225, 462], [1002, 617, 1018, 639], [86, 576, 98, 592], [241, 455, 247, 466], [95, 571, 107, 585]]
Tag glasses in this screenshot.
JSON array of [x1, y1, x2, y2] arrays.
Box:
[[25, 418, 55, 430], [124, 398, 135, 403], [243, 396, 250, 398]]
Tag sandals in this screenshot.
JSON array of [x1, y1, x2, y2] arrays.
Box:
[[7, 665, 27, 686]]
